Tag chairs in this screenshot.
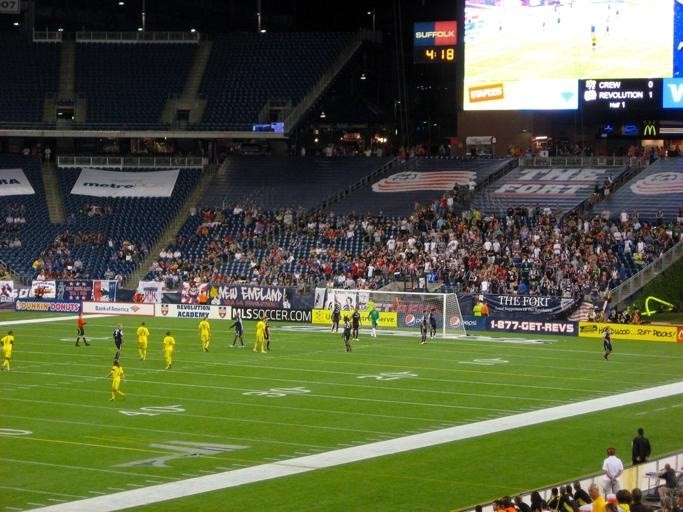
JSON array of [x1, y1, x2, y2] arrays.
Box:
[[0, 0, 681, 325]]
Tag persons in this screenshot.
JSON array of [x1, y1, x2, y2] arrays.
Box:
[[475, 427, 683, 512]]
